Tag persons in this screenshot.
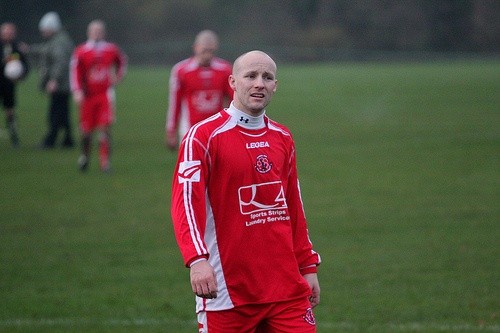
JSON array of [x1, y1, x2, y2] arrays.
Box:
[[167, 30, 234, 149], [35, 11, 75, 150], [171, 51, 320, 333], [0, 22, 31, 147], [69, 22, 126, 169]]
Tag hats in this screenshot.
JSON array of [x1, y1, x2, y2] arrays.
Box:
[[38, 12, 63, 34]]
